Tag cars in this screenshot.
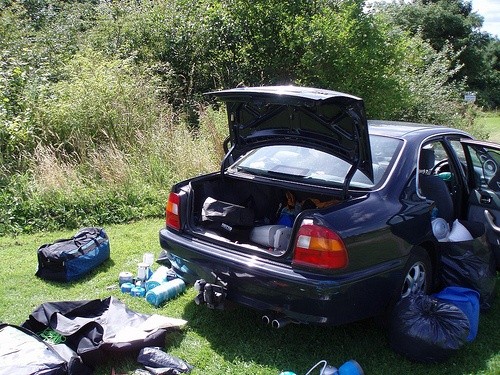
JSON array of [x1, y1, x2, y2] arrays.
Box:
[[159, 84, 500, 338]]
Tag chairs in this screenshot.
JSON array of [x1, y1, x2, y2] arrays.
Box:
[[419, 149, 454, 218]]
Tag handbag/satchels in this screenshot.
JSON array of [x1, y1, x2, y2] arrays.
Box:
[[423, 239, 498, 310], [392, 291, 470, 363], [35, 227, 111, 283], [435, 285, 482, 345]]
